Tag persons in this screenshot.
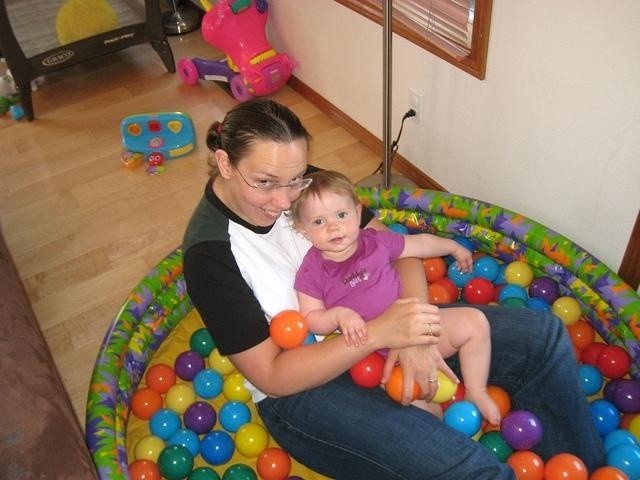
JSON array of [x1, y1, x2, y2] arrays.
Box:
[[181, 97, 611, 479], [284, 169, 506, 428]]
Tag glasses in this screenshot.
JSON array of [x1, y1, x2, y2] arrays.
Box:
[[230, 159, 314, 195]]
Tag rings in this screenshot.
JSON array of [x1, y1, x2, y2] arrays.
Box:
[[428, 324, 434, 337], [429, 378, 439, 385]]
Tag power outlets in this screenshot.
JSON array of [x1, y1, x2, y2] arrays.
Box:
[[405, 89, 425, 126]]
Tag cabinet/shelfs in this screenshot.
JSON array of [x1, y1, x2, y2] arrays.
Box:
[[1, 0, 179, 124]]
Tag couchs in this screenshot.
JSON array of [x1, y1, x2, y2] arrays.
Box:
[[2, 231, 105, 480]]
